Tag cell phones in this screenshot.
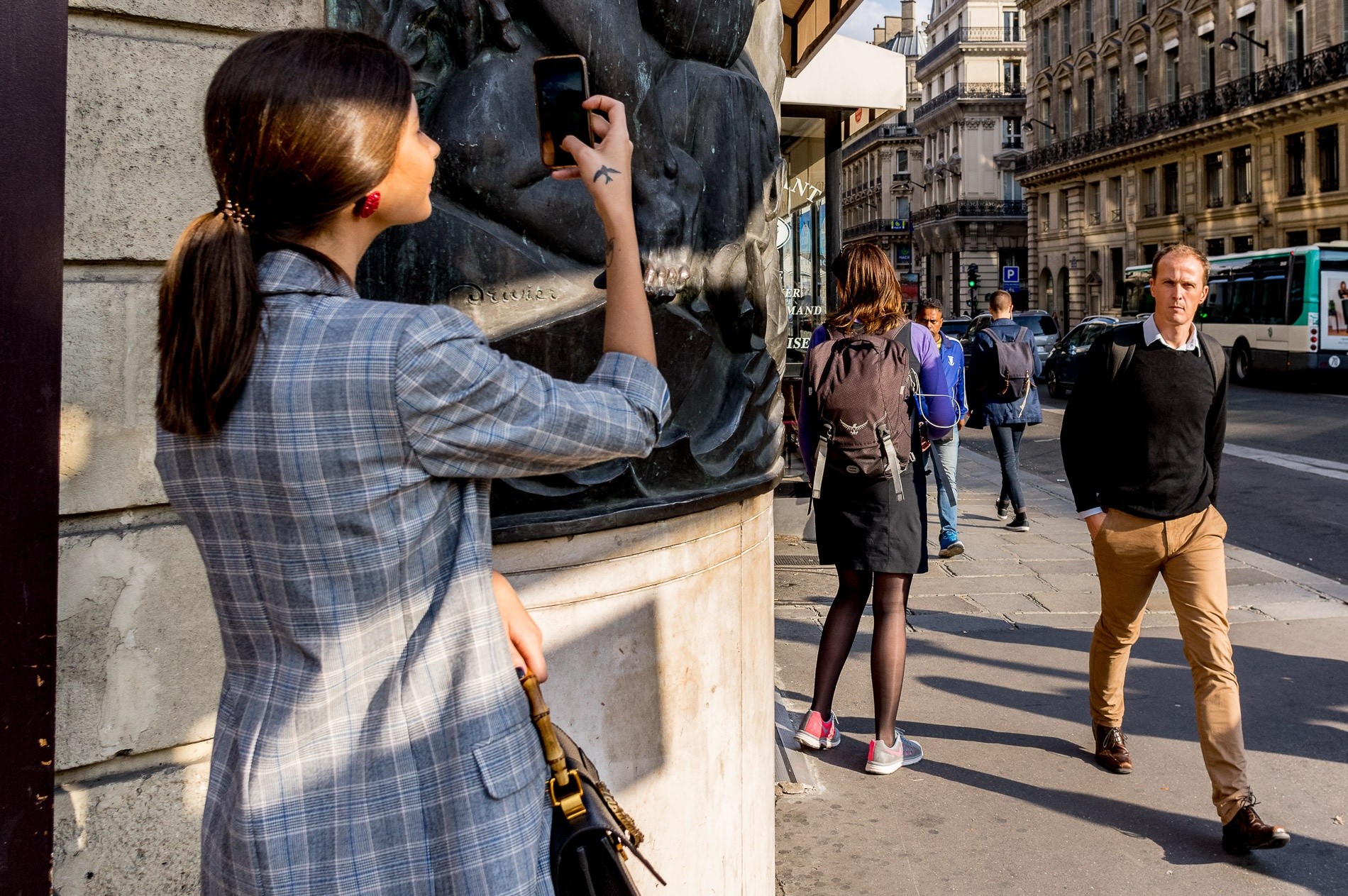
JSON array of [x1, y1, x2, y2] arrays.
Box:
[[533, 54, 595, 168]]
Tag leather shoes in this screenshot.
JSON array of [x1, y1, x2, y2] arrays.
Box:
[[1092, 719, 1133, 774], [1223, 785, 1291, 854]]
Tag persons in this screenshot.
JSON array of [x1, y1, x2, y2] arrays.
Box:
[[914, 297, 968, 559], [794, 241, 955, 775], [964, 290, 1044, 532], [1059, 244, 1291, 855], [1337, 281, 1348, 299], [151, 27, 673, 895]]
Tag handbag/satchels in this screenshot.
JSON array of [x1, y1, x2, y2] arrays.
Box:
[[521, 666, 668, 896]]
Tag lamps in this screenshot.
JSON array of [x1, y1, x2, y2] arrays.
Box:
[[934, 168, 962, 180], [1220, 32, 1268, 56], [902, 180, 926, 192], [856, 202, 877, 211], [1021, 119, 1056, 135]]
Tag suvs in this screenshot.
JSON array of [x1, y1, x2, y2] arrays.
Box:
[[960, 309, 1061, 372]]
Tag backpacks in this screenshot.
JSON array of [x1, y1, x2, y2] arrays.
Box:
[[808, 317, 914, 501], [979, 326, 1037, 398]]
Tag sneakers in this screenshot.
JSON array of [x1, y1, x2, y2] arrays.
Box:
[[938, 531, 965, 557], [1005, 511, 1030, 531], [865, 726, 924, 775], [995, 498, 1010, 519], [793, 708, 841, 749]]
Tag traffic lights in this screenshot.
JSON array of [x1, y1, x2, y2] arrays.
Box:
[[967, 263, 977, 288], [974, 274, 981, 290]]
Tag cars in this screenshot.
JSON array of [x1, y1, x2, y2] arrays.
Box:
[[788, 313, 816, 331], [1115, 239, 1348, 386], [1044, 315, 1145, 399], [941, 314, 972, 340]]
